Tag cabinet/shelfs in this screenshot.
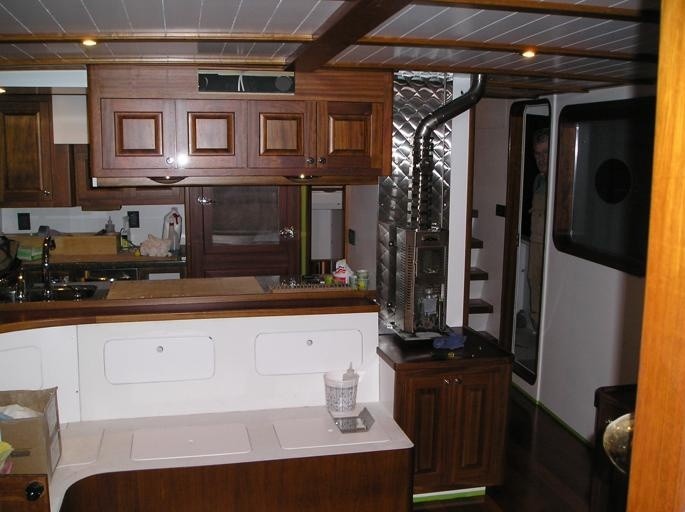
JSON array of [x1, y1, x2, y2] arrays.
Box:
[[21, 262, 185, 281], [376, 322, 515, 493]]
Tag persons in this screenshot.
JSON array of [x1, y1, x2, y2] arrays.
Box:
[[527, 127, 548, 332]]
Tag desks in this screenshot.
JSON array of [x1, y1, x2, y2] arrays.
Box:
[[589, 384, 637, 512]]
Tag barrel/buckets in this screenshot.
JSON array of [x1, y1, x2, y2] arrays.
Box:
[[162, 207, 182, 254]]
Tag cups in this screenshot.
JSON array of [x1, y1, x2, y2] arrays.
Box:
[[322, 369, 360, 415], [348, 270, 370, 290]]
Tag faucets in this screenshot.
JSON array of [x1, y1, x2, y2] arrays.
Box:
[[42, 234, 56, 299]]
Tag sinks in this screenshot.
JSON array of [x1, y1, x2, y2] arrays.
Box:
[[26, 282, 110, 301]]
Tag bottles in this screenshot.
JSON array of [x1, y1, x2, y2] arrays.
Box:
[[161, 207, 181, 253], [416, 287, 439, 332], [104, 215, 131, 250]]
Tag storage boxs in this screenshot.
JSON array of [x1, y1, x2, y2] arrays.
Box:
[[0, 385, 62, 481]]
